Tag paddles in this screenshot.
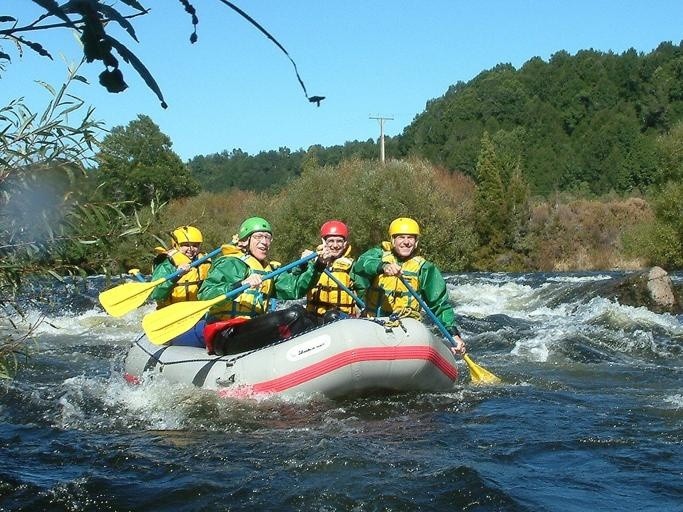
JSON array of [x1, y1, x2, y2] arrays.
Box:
[[396, 272, 501, 384], [142, 238, 329, 345], [98, 235, 238, 319]]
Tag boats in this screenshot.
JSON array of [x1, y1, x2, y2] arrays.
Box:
[[123, 317, 459, 400]]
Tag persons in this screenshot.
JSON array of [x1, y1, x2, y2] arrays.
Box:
[[194, 216, 334, 356], [294, 221, 364, 327], [348, 217, 466, 357], [148, 225, 240, 311]]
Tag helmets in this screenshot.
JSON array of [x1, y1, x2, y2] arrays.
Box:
[[237, 216, 271, 241], [172, 225, 203, 248], [387, 216, 421, 239], [320, 219, 348, 241]]
